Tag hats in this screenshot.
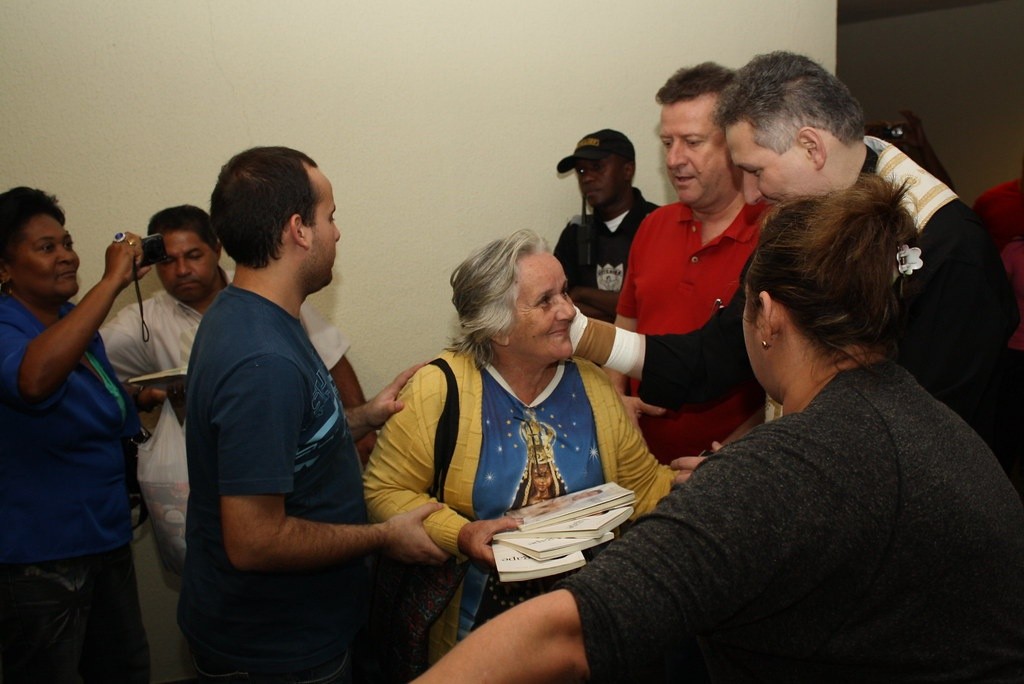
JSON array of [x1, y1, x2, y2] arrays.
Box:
[[557, 129, 635, 173]]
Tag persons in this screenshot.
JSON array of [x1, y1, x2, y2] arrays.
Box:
[[0, 186, 153, 684], [101, 206, 378, 584], [360, 229, 692, 670], [556, 51, 1024, 498], [173, 147, 448, 684], [524, 490, 604, 518], [409, 178, 1024, 684]]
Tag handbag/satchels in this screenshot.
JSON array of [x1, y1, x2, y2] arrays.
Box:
[[350, 549, 469, 684], [136, 396, 189, 573]]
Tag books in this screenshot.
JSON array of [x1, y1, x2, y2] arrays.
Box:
[[128, 365, 189, 387], [490, 481, 636, 584]]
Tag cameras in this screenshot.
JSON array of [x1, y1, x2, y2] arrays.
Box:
[[133, 233, 166, 269]]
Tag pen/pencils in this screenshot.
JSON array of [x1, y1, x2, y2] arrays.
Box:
[[710, 298, 724, 318]]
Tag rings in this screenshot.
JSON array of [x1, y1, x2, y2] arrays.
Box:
[[113, 232, 125, 242], [128, 239, 136, 247]]
